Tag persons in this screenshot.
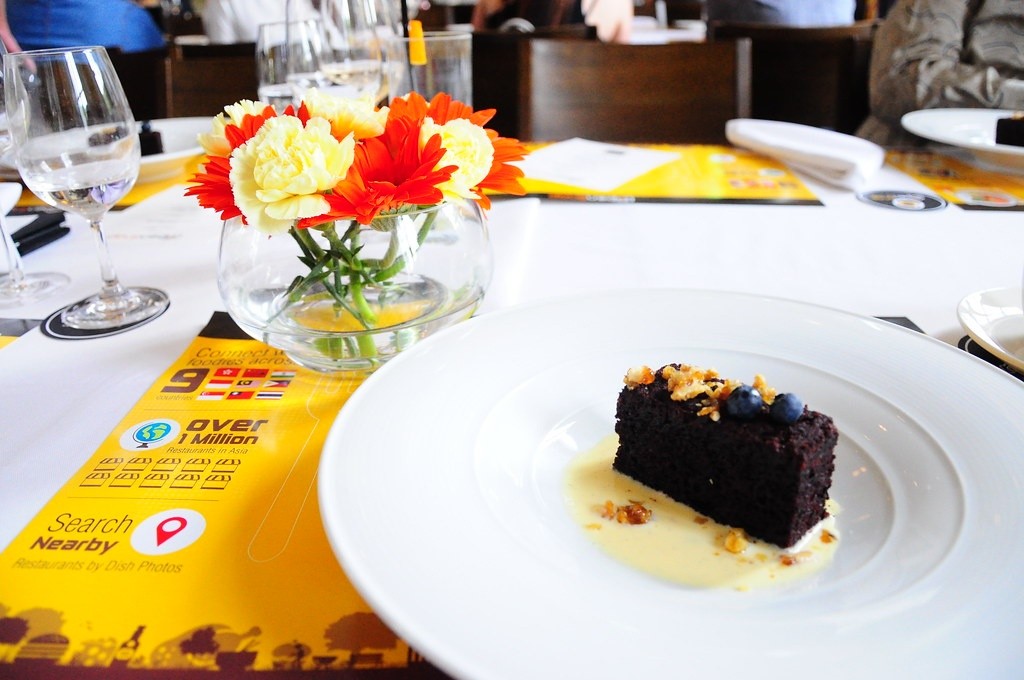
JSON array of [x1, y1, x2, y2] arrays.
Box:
[[0, 0, 167, 132], [854, 0, 1024, 147], [471, 0, 528, 32]]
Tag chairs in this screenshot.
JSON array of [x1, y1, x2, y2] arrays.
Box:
[[164, 59, 264, 119], [706, 19, 896, 139], [162, 41, 258, 59], [517, 34, 758, 149]]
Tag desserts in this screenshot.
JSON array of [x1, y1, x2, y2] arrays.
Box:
[[611, 363, 839, 549]]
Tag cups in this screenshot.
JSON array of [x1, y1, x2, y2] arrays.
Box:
[[256, 1, 475, 135]]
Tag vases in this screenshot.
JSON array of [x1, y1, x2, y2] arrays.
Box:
[[219, 195, 495, 377]]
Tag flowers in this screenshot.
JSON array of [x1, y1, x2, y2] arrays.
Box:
[[187, 91, 531, 237]]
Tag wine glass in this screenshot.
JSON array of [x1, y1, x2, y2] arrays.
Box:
[[0, 35, 170, 330]]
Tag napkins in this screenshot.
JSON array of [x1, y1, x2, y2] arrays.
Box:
[[724, 117, 894, 197]]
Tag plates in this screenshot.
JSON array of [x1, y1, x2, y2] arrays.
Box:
[[317, 291, 1023, 679], [955, 287, 1024, 372], [139, 118, 225, 181], [900, 109, 1024, 170]]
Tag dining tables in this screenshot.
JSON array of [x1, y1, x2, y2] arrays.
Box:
[[1, 118, 1024, 680]]
